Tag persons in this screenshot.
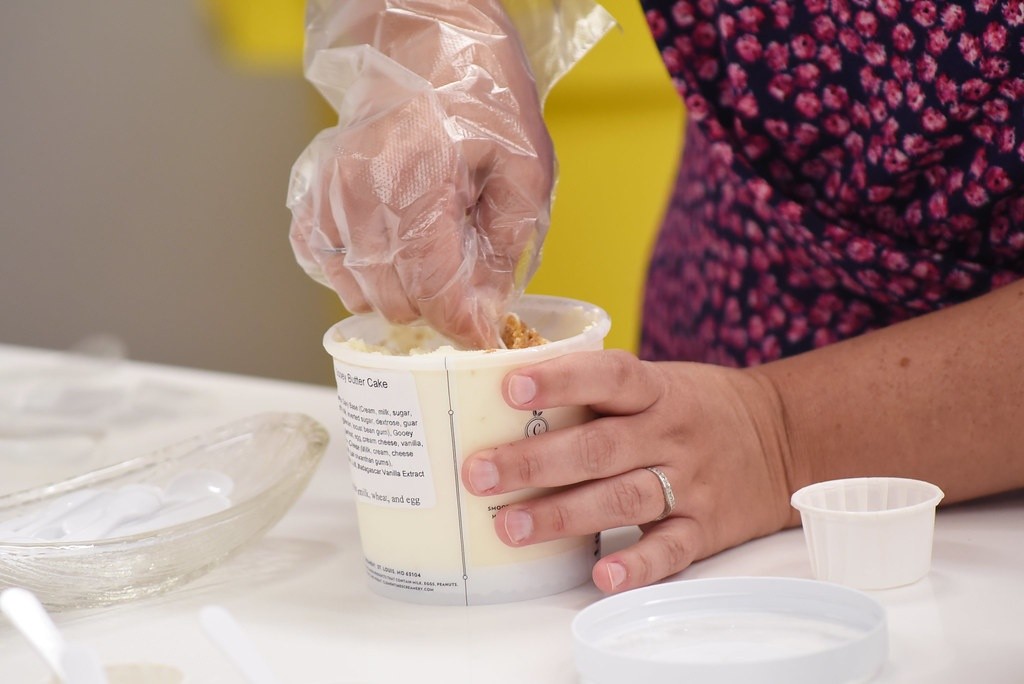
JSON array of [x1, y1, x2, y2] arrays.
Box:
[[287, 0, 1024, 595]]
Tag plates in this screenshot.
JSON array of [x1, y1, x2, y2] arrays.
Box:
[[568, 576, 889, 683], [0, 413, 329, 614]]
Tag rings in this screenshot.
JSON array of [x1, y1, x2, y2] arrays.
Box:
[[645, 466, 676, 522]]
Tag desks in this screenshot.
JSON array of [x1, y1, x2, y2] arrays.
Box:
[[0, 340, 1024, 684]]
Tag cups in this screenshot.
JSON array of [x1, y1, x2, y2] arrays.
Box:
[[322, 294, 614, 604], [790, 477, 945, 591]]
